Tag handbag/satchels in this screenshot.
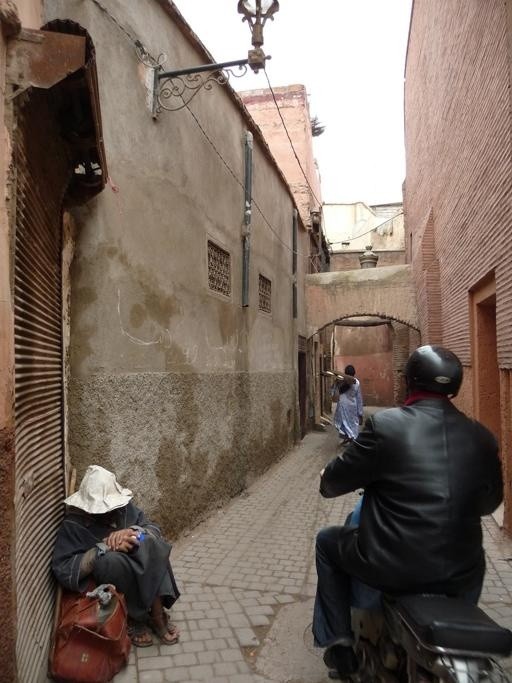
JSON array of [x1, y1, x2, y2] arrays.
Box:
[[47, 584, 132, 683]]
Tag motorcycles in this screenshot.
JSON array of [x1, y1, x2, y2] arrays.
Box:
[[330, 491, 512, 682]]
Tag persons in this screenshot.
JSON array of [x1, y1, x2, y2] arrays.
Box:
[[50, 461, 185, 646], [312, 344, 505, 676], [330, 364, 365, 445]]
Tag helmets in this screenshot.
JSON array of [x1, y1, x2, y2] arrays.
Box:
[[403, 345, 464, 398]]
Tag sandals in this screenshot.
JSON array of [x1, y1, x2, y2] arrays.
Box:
[[125, 620, 153, 648], [145, 611, 180, 645]]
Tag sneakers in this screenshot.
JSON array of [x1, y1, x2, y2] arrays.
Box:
[[323, 643, 360, 674], [342, 438, 352, 444]]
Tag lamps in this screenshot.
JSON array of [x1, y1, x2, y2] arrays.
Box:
[[150, 0, 286, 116]]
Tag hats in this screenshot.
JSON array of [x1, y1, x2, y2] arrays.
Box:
[[64, 464, 134, 515]]
